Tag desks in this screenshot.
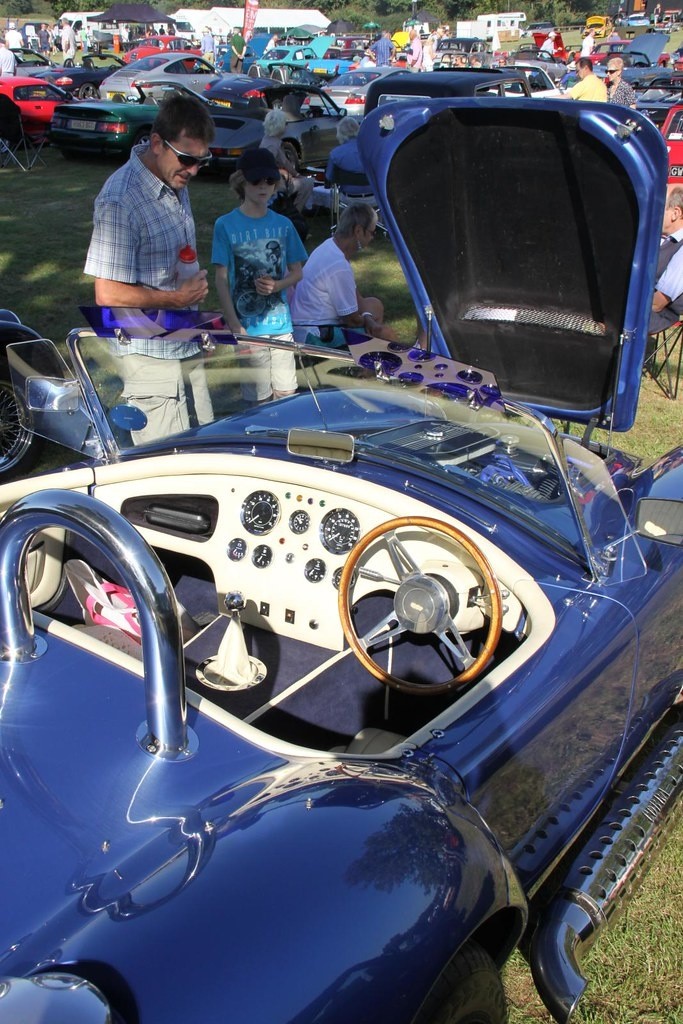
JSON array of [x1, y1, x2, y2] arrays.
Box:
[[311, 185, 335, 222]]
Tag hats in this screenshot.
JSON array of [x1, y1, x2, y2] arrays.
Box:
[[548, 32, 557, 37], [237, 148, 280, 182]]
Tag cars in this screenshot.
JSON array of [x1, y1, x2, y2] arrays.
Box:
[[0, 0, 683, 198], [1, 79, 683, 1024]]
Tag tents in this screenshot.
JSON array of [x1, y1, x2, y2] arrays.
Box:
[[85, 3, 175, 58], [404, 10, 441, 33]]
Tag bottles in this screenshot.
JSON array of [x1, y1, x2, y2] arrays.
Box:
[[175, 243, 200, 295]]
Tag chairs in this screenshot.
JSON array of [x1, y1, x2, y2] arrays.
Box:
[[247, 65, 260, 78], [84, 59, 94, 70], [0, 93, 48, 172], [65, 558, 200, 653], [329, 165, 381, 251], [269, 68, 287, 82]]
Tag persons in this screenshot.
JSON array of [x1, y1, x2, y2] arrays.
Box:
[[353, 19, 452, 73], [455, 55, 520, 93], [537, 27, 637, 110], [654, 4, 660, 28], [211, 148, 308, 408], [0, 17, 174, 77], [289, 202, 401, 355], [362, 311, 372, 318], [200, 26, 247, 75], [646, 182, 683, 339], [258, 110, 313, 215], [308, 115, 378, 208], [83, 96, 214, 447], [263, 33, 278, 60]]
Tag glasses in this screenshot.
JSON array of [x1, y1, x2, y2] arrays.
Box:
[[352, 223, 376, 237], [163, 140, 212, 168], [605, 69, 618, 74], [248, 178, 277, 186], [575, 69, 580, 73]]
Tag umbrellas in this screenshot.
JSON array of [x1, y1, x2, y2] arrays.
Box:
[[281, 24, 323, 40], [405, 19, 422, 26], [326, 20, 355, 34], [363, 21, 380, 42]]
